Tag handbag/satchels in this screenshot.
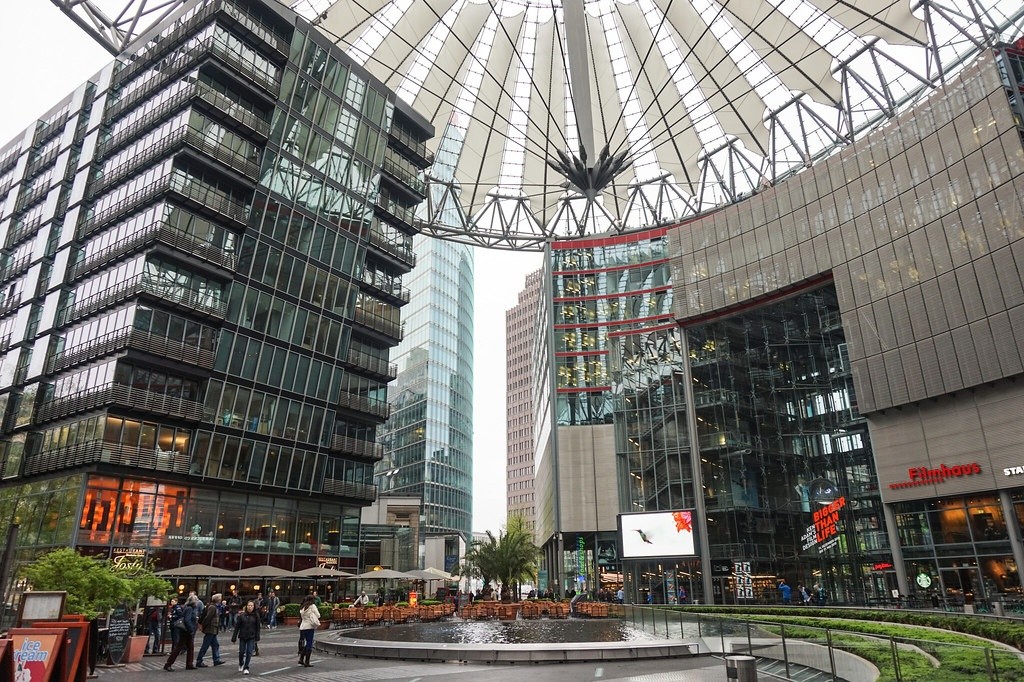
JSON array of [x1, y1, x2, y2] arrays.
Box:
[[174, 606, 187, 632], [309, 604, 321, 627]]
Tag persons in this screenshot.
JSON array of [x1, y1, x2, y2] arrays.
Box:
[[163, 595, 198, 672], [231, 600, 261, 675], [195, 593, 225, 667], [297, 595, 321, 668], [135, 579, 830, 658]]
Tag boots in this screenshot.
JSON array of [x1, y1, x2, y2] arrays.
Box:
[[298, 653, 305, 665], [304, 651, 315, 667]]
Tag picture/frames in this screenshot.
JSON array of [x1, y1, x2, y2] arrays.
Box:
[[0, 614, 90, 682]]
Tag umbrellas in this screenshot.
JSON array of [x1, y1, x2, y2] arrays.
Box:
[[152, 563, 458, 600]]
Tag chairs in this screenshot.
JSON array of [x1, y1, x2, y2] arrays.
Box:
[[899, 595, 1024, 614], [462, 600, 625, 619], [333, 603, 455, 630]]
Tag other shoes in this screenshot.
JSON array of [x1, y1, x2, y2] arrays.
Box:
[[164, 666, 176, 672], [214, 660, 225, 666], [196, 663, 208, 667], [243, 669, 249, 675], [238, 665, 244, 671], [255, 647, 259, 656], [186, 666, 198, 670]]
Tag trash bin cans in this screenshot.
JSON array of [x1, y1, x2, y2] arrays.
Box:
[[725, 656, 757, 682], [570, 594, 588, 618], [693, 599, 699, 604], [931, 593, 940, 609], [992, 602, 1006, 616]]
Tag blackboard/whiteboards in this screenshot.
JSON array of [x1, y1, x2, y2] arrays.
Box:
[[31, 622, 91, 682], [108, 616, 131, 665]]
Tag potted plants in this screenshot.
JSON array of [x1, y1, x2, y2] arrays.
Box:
[[326, 606, 332, 629], [117, 575, 167, 662], [316, 606, 327, 630], [283, 604, 301, 625]]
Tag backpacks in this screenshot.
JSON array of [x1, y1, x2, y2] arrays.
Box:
[[198, 604, 214, 624]]
